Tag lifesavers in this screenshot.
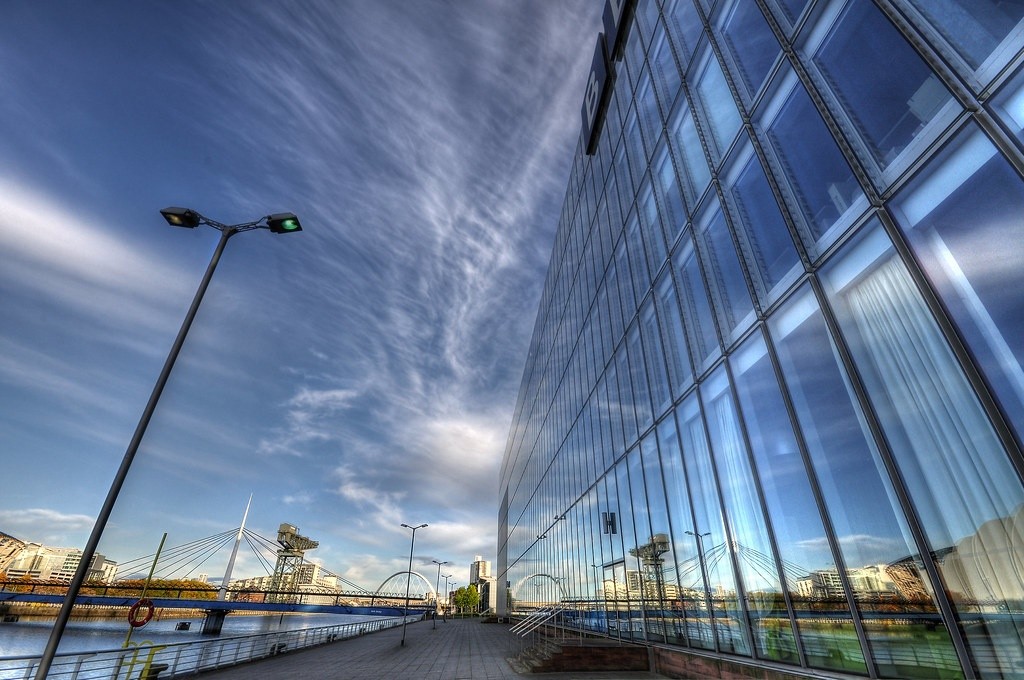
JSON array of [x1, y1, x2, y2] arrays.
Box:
[[128, 597, 155, 627]]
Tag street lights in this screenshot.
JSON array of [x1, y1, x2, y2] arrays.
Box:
[[449, 582, 457, 618], [590, 564, 602, 623], [685, 529, 721, 633], [34, 205, 303, 679], [399, 522, 428, 644], [432, 561, 448, 629], [441, 575, 453, 622]]
